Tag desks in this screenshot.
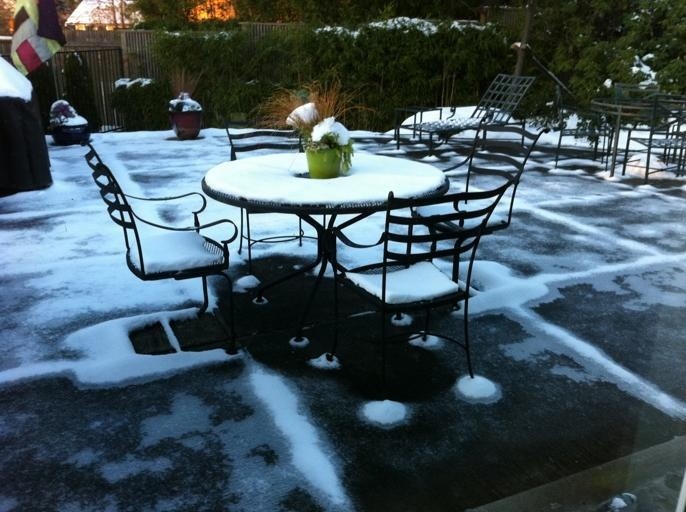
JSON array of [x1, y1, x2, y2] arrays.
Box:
[[202, 151, 449, 347]]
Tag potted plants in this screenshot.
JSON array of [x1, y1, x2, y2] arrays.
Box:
[[49, 99, 91, 144], [169, 93, 203, 138], [251, 117, 373, 179]]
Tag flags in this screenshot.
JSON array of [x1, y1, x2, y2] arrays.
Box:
[[9, 0, 67, 78]]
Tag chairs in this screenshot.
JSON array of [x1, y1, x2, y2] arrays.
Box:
[[396, 73, 537, 157], [224, 113, 326, 276], [81, 137, 239, 357], [326, 168, 522, 390], [405, 113, 550, 294], [556, 83, 686, 184]]
